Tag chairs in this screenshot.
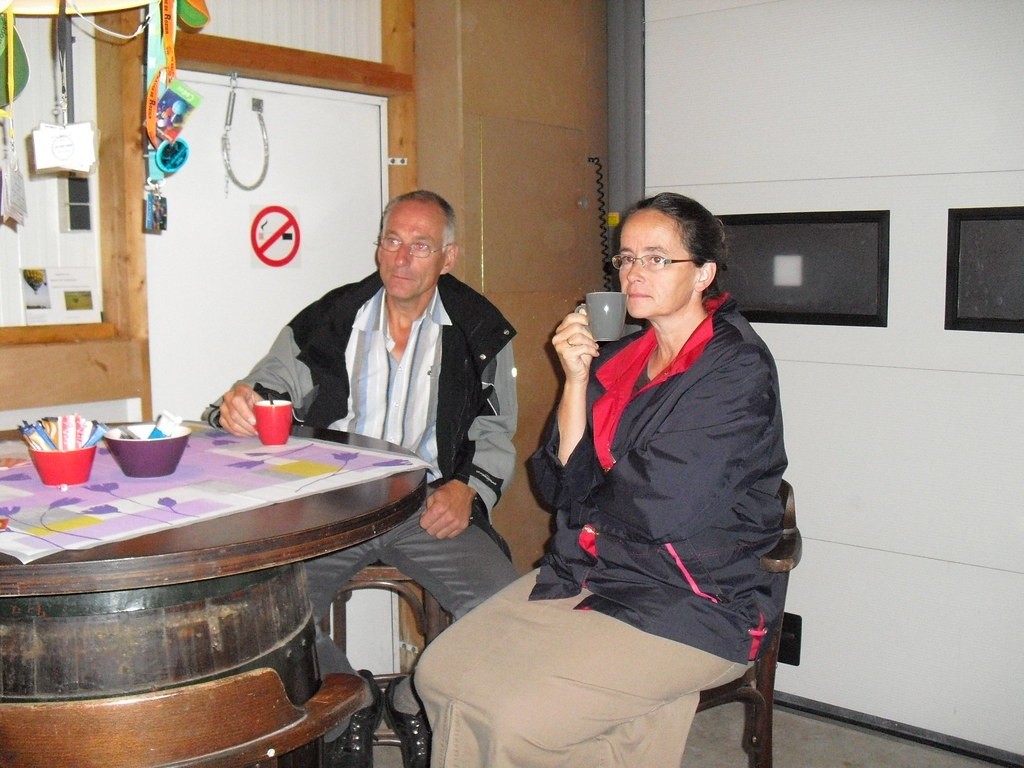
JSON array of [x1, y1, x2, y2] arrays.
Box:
[[695, 478, 798, 768], [321, 561, 455, 747]]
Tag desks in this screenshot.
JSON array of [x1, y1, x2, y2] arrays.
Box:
[[0, 420, 428, 768]]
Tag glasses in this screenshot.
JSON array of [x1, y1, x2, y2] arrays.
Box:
[[611, 254, 704, 273], [378, 232, 450, 259]]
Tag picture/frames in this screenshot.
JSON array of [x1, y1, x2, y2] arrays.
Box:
[[944, 206, 1024, 334], [714, 208, 890, 328]]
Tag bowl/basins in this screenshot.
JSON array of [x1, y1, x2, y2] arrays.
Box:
[[103, 423, 193, 478], [29, 445, 97, 487]]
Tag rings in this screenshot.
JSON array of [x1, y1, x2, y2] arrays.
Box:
[[567, 339, 576, 346]]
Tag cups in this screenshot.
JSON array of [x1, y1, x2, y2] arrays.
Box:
[[574, 290, 628, 341], [252, 399, 294, 446]]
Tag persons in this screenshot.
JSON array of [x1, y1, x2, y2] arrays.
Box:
[[204, 189, 519, 768], [413, 194, 789, 768]]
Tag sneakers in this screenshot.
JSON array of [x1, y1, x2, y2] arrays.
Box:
[[384, 675, 432, 768], [322, 669, 384, 768]]
